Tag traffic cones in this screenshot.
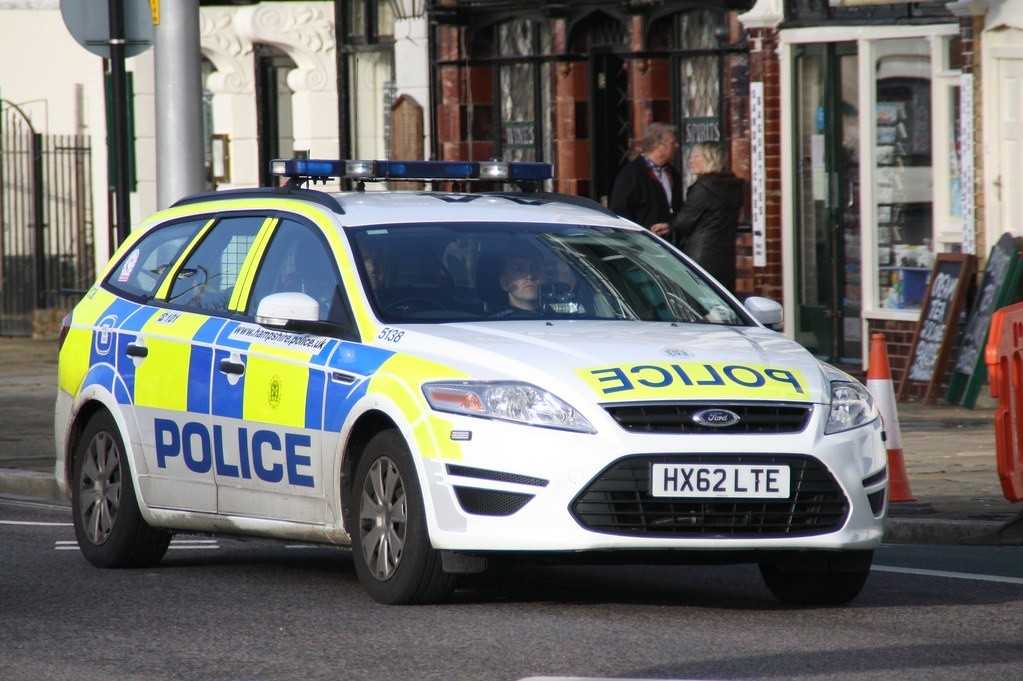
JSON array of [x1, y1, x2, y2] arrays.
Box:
[[867, 332, 917, 503]]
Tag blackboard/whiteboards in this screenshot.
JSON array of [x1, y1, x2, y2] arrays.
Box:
[[951, 231, 1023, 376], [907, 251, 978, 381]]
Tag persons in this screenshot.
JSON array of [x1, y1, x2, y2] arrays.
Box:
[[498, 250, 556, 318], [611, 124, 679, 233], [338, 254, 390, 316], [653, 140, 743, 288]]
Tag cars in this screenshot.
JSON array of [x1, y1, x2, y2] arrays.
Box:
[[54, 158, 890, 605]]
